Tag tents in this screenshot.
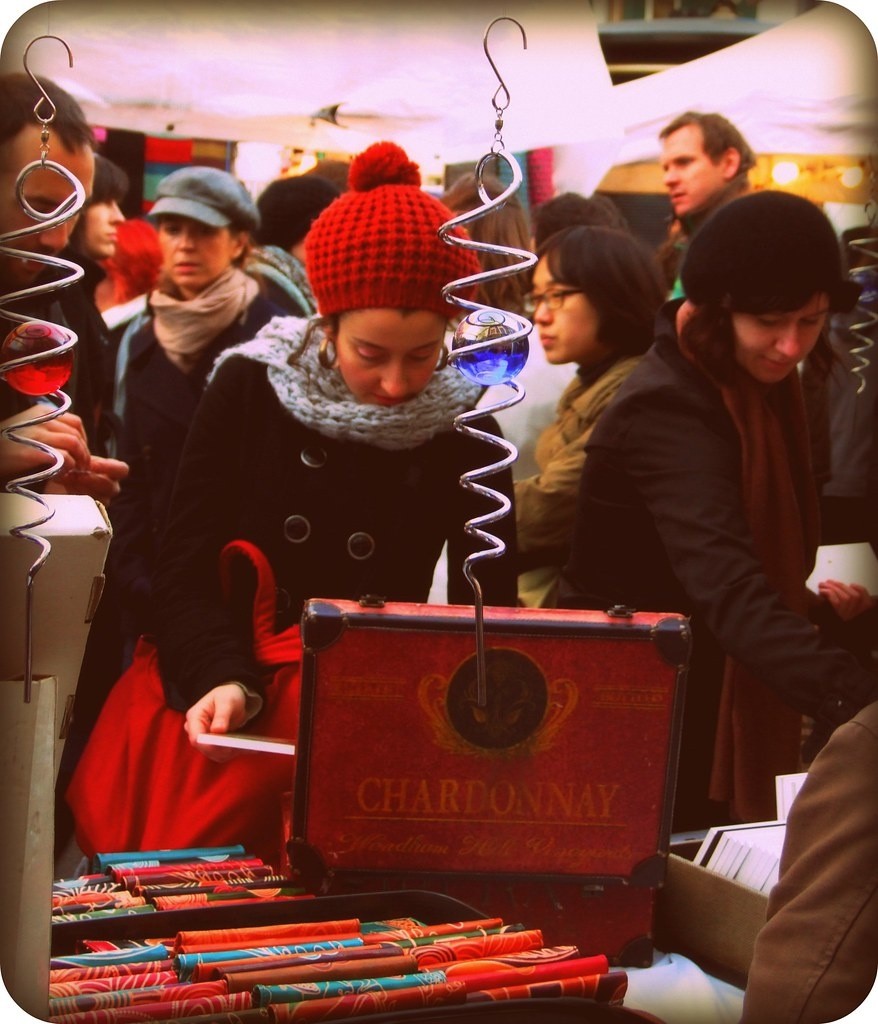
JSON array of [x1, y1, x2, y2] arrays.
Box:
[[1, 0, 613, 167], [613, 4, 877, 205]]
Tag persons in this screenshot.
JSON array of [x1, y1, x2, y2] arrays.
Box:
[[1, 75, 670, 856], [653, 110, 757, 251], [737, 701, 877, 1024], [569, 190, 878, 829]]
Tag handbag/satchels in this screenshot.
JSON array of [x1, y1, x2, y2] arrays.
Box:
[[67, 540, 300, 859]]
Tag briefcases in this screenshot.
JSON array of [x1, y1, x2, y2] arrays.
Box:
[[288, 594, 691, 966]]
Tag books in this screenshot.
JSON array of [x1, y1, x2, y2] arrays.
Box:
[[695, 820, 786, 893]]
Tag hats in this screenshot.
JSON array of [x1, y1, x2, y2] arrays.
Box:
[[304, 141, 482, 319], [147, 166, 262, 234], [681, 192, 843, 299]]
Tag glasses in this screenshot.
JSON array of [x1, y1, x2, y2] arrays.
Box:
[[521, 287, 584, 312]]
